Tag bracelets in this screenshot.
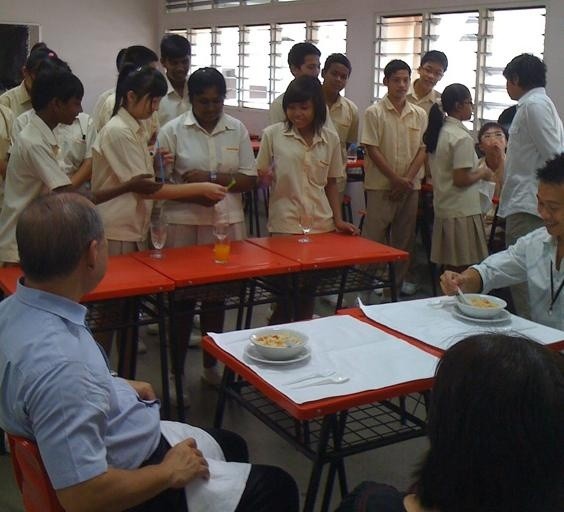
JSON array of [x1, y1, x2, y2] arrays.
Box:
[[211, 171, 217, 184], [208, 171, 212, 182]]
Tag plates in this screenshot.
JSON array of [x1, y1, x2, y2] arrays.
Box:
[[452, 305, 511, 323], [244, 342, 311, 364]]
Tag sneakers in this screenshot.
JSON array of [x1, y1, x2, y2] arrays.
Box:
[[200, 367, 224, 390]]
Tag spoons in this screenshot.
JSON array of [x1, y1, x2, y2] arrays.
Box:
[[295, 375, 349, 386], [456, 285, 472, 306]]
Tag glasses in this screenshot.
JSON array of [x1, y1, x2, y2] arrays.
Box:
[[463, 101, 473, 106], [480, 130, 506, 140]]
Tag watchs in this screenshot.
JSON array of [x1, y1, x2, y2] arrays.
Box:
[[109, 369, 118, 377]]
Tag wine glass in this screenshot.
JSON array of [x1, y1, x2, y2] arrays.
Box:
[[299, 214, 313, 244], [150, 216, 168, 259], [212, 207, 232, 243]]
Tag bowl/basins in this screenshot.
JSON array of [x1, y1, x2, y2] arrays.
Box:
[[248, 327, 308, 361], [456, 292, 507, 318]]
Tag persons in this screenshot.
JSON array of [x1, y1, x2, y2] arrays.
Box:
[[316, 53, 359, 309], [503, 53, 564, 322], [422, 83, 489, 274], [256, 74, 361, 326], [0, 193, 300, 512], [146, 34, 204, 349], [374, 50, 448, 296], [7, 56, 95, 195], [90, 63, 228, 380], [477, 123, 505, 254], [266, 42, 321, 320], [0, 71, 165, 300], [439, 151, 564, 331], [0, 43, 58, 117], [0, 104, 18, 211], [155, 68, 259, 409], [96, 45, 159, 354], [331, 332, 564, 512], [345, 60, 429, 307]]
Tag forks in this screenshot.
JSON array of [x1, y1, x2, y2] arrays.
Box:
[[283, 370, 335, 384]]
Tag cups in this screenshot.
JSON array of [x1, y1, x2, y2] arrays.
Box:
[[213, 229, 232, 264], [346, 145, 358, 165]]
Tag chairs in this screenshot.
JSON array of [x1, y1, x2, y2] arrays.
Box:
[[5, 433, 64, 512]]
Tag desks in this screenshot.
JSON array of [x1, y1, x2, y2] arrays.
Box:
[[235, 230, 409, 394], [0, 252, 171, 421], [198, 314, 441, 512], [337, 294, 563, 352], [129, 238, 300, 412]]
